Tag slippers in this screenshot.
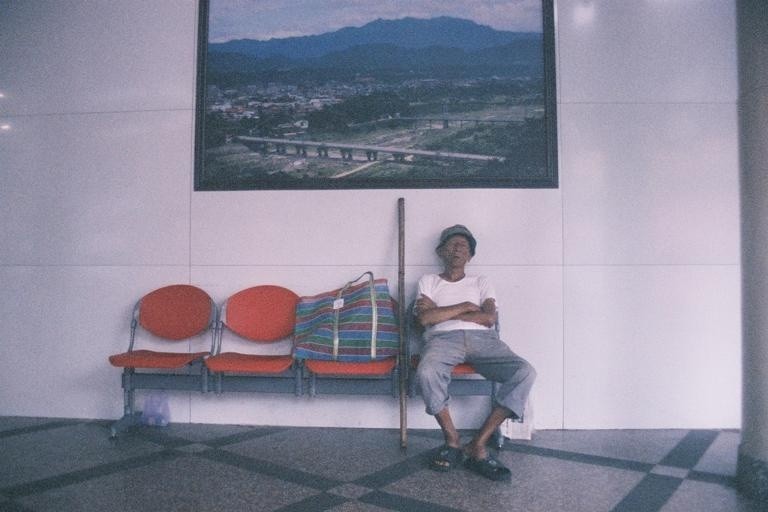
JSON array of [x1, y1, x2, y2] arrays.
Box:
[[429, 444, 464, 472], [465, 456, 512, 481]]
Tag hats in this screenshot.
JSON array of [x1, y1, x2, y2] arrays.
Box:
[[435, 224, 476, 257]]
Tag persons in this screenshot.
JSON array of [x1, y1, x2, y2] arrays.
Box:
[[412, 225, 537, 480]]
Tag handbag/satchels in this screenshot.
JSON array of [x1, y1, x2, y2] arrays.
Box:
[[293, 279, 400, 362]]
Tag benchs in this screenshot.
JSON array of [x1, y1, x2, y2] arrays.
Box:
[[107, 284, 510, 452]]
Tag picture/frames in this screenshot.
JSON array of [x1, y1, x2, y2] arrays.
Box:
[[192, 0, 558, 191]]
